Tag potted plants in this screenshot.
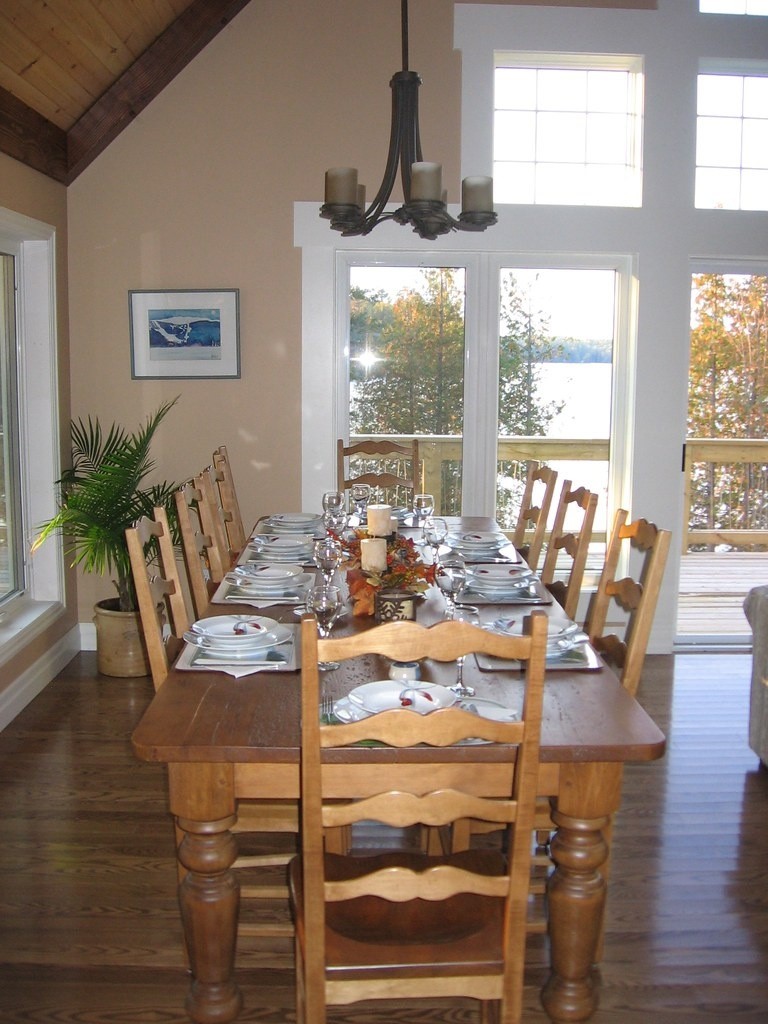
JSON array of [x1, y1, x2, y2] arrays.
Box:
[[28, 392, 199, 677]]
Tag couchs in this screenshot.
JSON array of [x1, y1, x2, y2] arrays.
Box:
[[743, 585, 768, 774]]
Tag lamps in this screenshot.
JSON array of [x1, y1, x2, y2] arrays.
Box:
[[319, 1, 498, 240]]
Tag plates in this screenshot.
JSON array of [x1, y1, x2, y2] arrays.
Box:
[[333, 695, 372, 725], [181, 512, 323, 655], [293, 604, 348, 617], [445, 530, 590, 658], [347, 680, 457, 713]]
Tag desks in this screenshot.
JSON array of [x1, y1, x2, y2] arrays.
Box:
[[132, 516, 667, 1024]]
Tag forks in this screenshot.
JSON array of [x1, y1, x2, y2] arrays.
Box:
[[321, 695, 334, 725]]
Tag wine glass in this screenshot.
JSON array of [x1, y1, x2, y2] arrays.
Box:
[[314, 491, 348, 587], [437, 559, 468, 620], [443, 605, 481, 696], [351, 483, 371, 525], [413, 494, 435, 545], [306, 586, 343, 639], [423, 518, 447, 564]]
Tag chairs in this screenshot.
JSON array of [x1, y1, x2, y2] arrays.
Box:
[[126, 444, 352, 975], [286, 610, 548, 1024], [337, 439, 421, 511], [417, 459, 672, 963]]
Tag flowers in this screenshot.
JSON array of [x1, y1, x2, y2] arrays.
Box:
[[326, 527, 438, 615]]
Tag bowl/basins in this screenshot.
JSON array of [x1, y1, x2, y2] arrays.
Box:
[[389, 662, 421, 680]]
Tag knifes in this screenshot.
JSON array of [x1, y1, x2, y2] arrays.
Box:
[[470, 703, 479, 718]]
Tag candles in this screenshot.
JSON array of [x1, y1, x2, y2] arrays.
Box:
[[380, 594, 413, 620], [359, 538, 387, 572], [391, 516, 397, 532], [367, 504, 392, 536]]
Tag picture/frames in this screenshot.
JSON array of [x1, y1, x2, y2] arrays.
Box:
[[128, 288, 241, 381]]
